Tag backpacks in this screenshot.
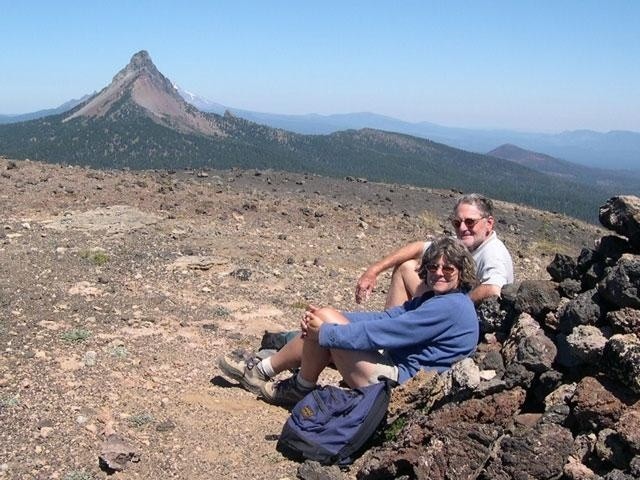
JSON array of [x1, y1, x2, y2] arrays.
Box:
[[276, 380, 390, 468]]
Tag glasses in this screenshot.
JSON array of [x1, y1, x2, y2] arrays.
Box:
[[425, 264, 458, 274], [451, 216, 485, 229]]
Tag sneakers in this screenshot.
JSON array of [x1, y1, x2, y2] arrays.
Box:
[[260, 330, 293, 349], [218, 348, 318, 406]]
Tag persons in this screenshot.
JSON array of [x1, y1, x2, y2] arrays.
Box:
[[221, 238, 478, 406], [259, 194, 513, 349]]
